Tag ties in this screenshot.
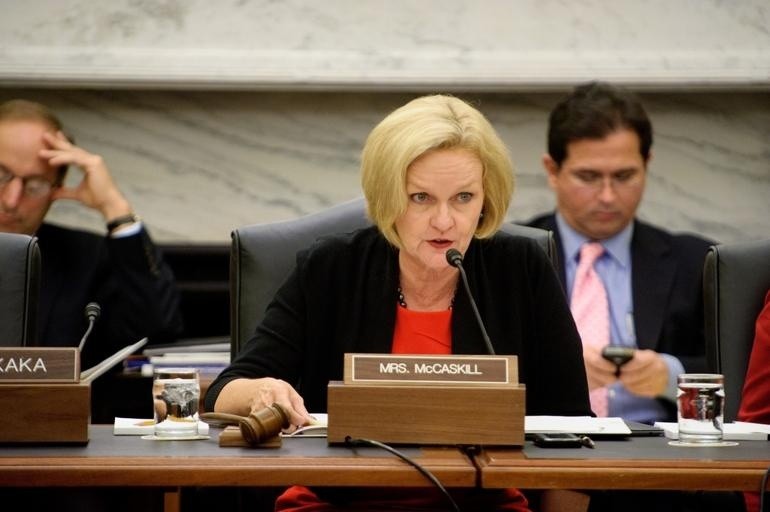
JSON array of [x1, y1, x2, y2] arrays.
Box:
[[571, 242, 611, 418]]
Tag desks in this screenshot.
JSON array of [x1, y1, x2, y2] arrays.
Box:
[[0, 416, 769, 512]]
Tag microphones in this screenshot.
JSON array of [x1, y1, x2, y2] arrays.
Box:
[[77, 302, 102, 352], [445, 248, 497, 355]]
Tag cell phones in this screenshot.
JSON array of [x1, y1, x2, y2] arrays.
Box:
[[602, 345, 634, 365], [536, 433, 582, 449]]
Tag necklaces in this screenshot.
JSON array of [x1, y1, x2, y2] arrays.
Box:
[[397, 288, 457, 311]]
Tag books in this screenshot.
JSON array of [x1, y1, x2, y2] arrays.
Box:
[[654, 421, 770, 440]]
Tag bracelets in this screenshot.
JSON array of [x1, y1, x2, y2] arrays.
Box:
[[107, 213, 137, 231]]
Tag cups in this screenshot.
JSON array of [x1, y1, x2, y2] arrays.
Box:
[[153, 367, 201, 435], [676, 372, 726, 443]]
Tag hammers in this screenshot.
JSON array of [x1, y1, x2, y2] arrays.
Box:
[[199, 402, 290, 443]]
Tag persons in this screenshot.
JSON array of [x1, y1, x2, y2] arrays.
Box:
[[509, 81, 720, 425], [203, 94, 597, 512], [737, 289, 770, 512], [0, 99, 184, 512]]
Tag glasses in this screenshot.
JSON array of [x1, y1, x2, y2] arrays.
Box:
[[0, 167, 54, 202]]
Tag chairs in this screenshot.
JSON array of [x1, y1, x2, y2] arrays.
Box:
[[230, 195, 560, 363], [701, 236, 770, 423], [0, 231, 41, 347]]
